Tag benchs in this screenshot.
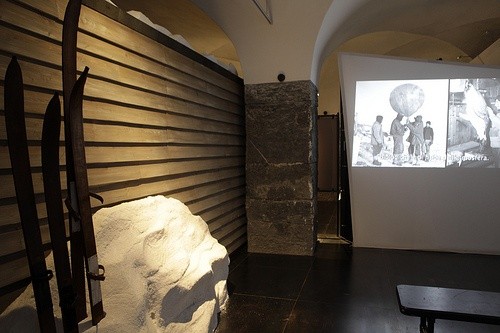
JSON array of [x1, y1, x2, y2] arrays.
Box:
[[396, 285, 500, 333]]
